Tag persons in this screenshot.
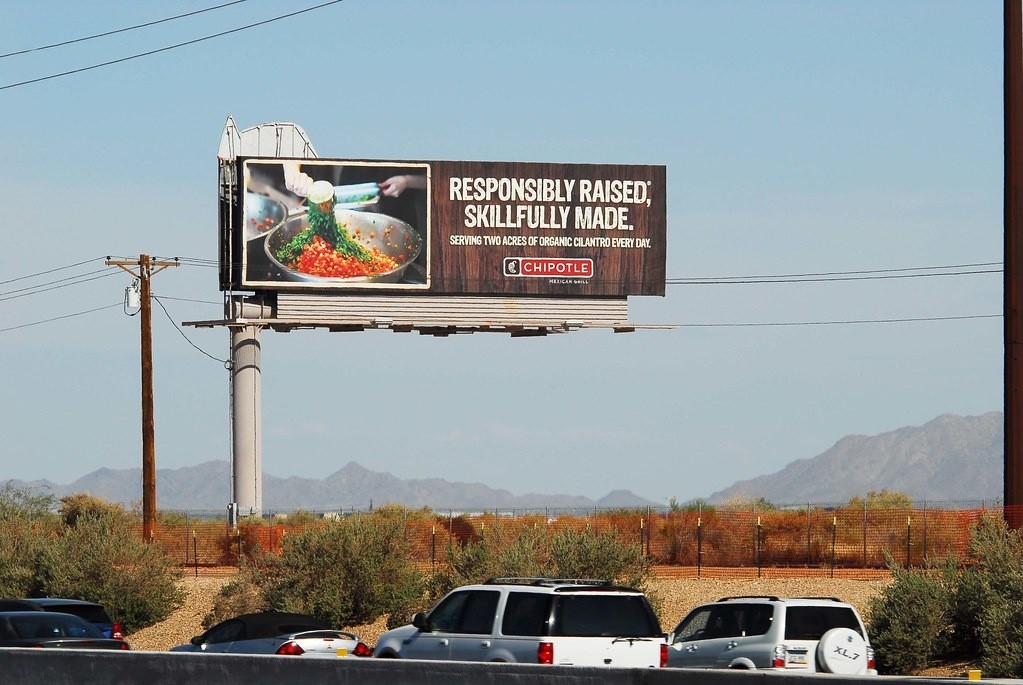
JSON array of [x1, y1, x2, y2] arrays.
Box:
[[283, 164, 428, 232]]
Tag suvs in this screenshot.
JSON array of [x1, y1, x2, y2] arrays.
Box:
[[669, 596, 878, 675], [373, 577, 668, 669]]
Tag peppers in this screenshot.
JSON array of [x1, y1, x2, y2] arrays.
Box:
[[250, 215, 412, 278]]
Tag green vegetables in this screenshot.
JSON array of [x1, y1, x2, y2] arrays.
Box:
[[277, 192, 372, 262]]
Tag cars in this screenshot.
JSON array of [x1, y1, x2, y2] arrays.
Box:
[[0, 612, 130, 650], [169, 611, 373, 658], [0, 598, 123, 641]]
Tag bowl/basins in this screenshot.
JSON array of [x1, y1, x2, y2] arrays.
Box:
[[245, 191, 287, 258], [264, 210, 423, 288]]
